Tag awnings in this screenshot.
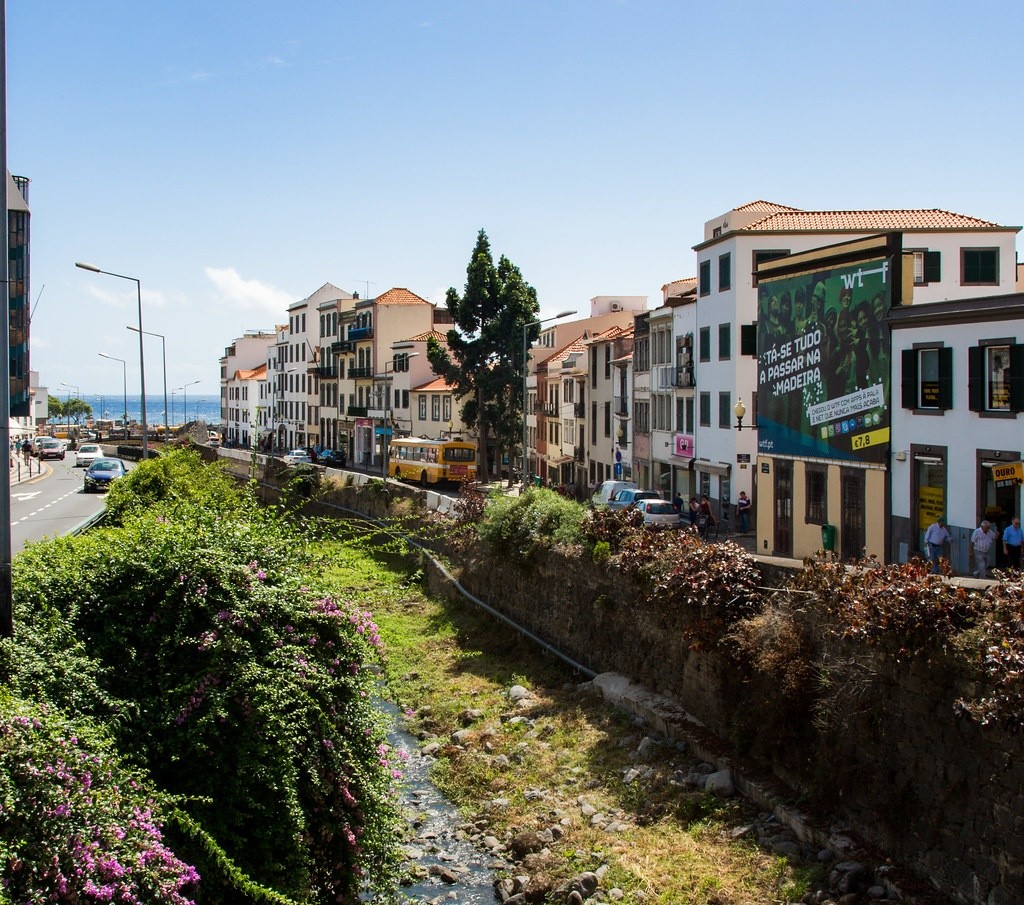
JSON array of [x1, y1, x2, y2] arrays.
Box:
[[260, 429, 275, 438]]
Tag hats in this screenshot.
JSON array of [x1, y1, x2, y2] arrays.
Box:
[[938, 517, 947, 523]]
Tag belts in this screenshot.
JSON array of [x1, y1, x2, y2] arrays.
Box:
[[929, 542, 942, 547]]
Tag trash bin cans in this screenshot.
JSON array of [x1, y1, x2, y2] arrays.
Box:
[[534, 476, 541, 486], [821, 524, 836, 551]]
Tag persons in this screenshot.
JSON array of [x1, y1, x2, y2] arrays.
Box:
[[589, 480, 603, 490], [736, 491, 752, 534], [675, 493, 685, 511], [9, 435, 33, 466], [689, 495, 713, 533], [547, 481, 583, 503], [923, 517, 953, 574], [70, 427, 78, 442], [1002, 517, 1024, 572], [968, 520, 1000, 579]]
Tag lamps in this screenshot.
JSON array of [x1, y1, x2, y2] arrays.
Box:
[[342, 411, 354, 424], [446, 420, 468, 435], [733, 397, 758, 431], [615, 427, 631, 446]]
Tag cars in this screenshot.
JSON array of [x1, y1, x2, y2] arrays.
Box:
[[206, 436, 220, 448], [630, 497, 681, 531], [83, 457, 130, 493], [22, 417, 125, 461], [282, 446, 348, 468], [74, 443, 105, 468]]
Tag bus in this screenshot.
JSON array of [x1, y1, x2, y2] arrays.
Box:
[[388, 435, 478, 488]]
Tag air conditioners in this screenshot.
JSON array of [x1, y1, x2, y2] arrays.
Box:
[[611, 302, 621, 312]]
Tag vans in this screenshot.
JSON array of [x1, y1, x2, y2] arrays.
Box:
[[590, 479, 639, 508]]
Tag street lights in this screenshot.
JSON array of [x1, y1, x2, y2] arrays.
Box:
[[75, 260, 149, 460], [197, 399, 207, 421], [183, 380, 202, 433], [126, 325, 169, 448], [270, 367, 298, 468], [94, 393, 106, 419], [171, 387, 185, 445], [99, 352, 128, 446], [73, 391, 85, 440], [57, 388, 70, 444], [382, 351, 421, 490], [523, 308, 580, 483], [60, 382, 80, 444]]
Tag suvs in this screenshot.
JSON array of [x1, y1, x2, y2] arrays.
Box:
[[605, 489, 662, 511]]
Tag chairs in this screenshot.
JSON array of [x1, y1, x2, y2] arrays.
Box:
[[97, 464, 118, 469]]
